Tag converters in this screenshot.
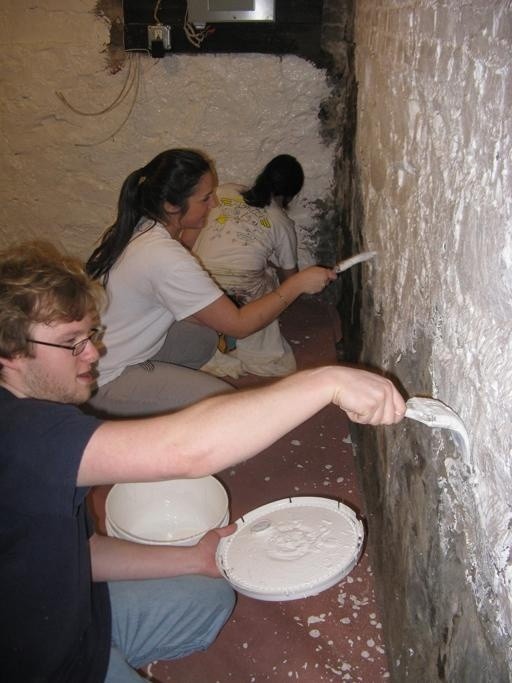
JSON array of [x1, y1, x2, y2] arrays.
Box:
[[150, 38, 165, 59]]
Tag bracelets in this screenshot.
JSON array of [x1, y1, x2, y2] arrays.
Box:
[[272, 290, 288, 307]]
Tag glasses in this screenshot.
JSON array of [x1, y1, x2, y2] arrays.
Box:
[[27, 325, 105, 356]]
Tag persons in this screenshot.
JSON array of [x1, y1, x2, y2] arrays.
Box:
[[180, 154, 304, 379], [87, 148, 338, 416], [0, 240, 407, 683]]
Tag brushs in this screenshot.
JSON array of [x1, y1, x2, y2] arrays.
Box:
[[333, 250, 377, 273], [339, 399, 463, 430]]
